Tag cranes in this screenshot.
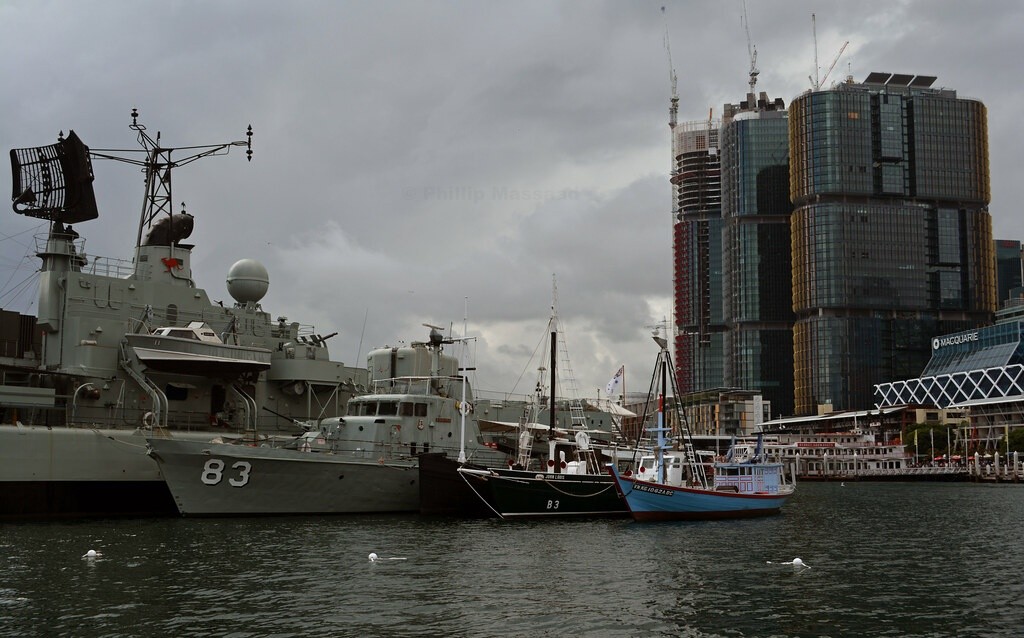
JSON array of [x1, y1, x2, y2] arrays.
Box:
[[808, 13, 851, 92], [660, 5, 680, 227], [739, 1, 761, 93]]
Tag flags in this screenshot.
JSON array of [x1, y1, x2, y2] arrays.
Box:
[[606, 366, 623, 395]]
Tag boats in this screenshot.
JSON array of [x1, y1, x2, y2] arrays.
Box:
[[79, 388, 101, 401], [0, 103, 634, 525]]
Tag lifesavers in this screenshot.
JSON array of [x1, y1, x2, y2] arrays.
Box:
[[143, 412, 159, 429]]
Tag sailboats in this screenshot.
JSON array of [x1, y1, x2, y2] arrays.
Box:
[[610, 312, 794, 522]]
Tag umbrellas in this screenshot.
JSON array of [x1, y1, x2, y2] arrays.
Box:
[[934, 454, 1004, 460]]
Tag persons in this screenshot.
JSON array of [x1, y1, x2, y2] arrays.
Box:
[[908, 460, 1024, 470]]
